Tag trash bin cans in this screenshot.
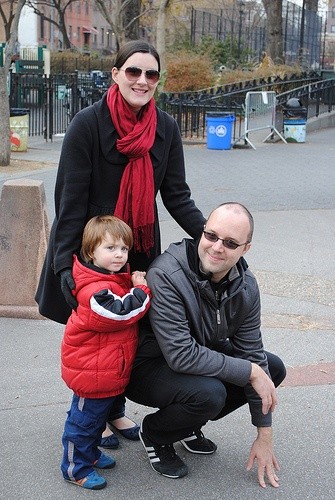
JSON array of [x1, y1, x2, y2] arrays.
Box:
[[283, 98, 308, 143], [9, 107, 30, 151], [205, 112, 235, 149]]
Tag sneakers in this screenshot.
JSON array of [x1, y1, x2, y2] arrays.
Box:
[[93, 451, 116, 469], [65, 471, 107, 489], [139, 429, 188, 479], [179, 429, 217, 454]]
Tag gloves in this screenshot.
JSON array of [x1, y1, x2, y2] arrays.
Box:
[[60, 268, 78, 309], [236, 257, 248, 277]]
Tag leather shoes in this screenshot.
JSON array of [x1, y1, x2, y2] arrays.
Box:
[[107, 415, 141, 441], [99, 433, 119, 449]]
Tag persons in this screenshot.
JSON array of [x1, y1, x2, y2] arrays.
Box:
[[34, 42, 206, 450], [123, 202, 286, 488], [61, 216, 153, 491]]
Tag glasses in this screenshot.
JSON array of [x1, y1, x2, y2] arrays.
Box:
[[203, 230, 247, 249], [118, 67, 160, 84]]
